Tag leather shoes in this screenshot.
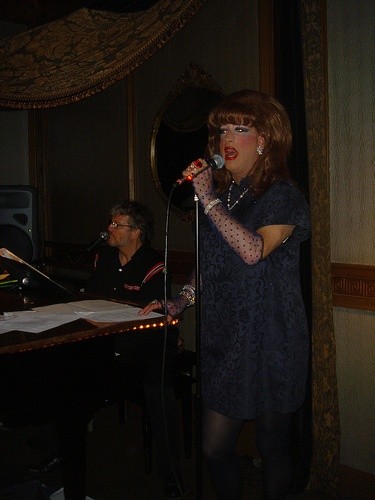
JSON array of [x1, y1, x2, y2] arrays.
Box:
[[0, 412, 32, 431], [24, 452, 64, 477]]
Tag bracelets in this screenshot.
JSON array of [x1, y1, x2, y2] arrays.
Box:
[[204, 198, 222, 216], [179, 285, 196, 307]]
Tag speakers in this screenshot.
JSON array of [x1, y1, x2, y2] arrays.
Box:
[[0, 185, 39, 269]]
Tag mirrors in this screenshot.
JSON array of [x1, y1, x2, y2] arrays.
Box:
[[148, 60, 228, 213]]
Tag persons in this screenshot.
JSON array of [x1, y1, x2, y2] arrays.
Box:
[[98, 200, 171, 316], [138, 90, 313, 500]]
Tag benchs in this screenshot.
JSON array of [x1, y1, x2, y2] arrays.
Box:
[[36, 239, 196, 459]]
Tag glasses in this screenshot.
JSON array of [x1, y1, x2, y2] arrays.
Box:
[[107, 219, 137, 230]]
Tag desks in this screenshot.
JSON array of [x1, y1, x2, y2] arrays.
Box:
[[0, 290, 184, 500]]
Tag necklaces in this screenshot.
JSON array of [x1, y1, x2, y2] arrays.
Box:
[[227, 179, 254, 212]]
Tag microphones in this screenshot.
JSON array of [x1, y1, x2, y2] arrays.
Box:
[[172, 153, 225, 189], [80, 231, 108, 258]]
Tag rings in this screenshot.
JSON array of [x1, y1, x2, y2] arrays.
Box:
[[187, 159, 206, 173], [151, 299, 159, 303]]
